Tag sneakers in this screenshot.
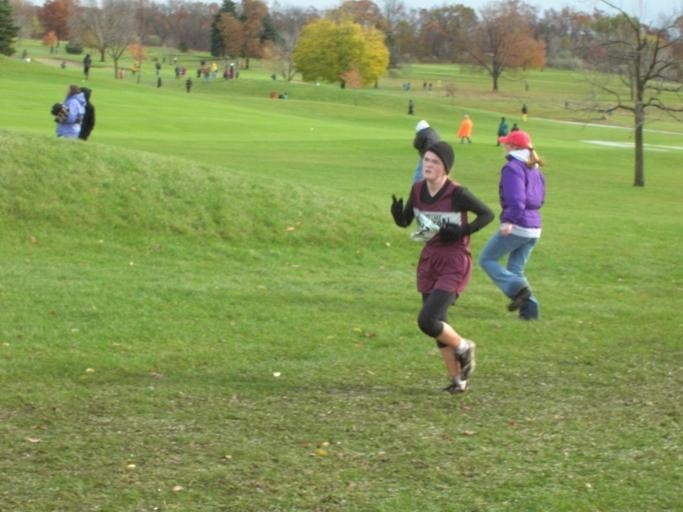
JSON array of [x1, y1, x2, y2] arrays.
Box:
[[456, 339, 476, 381], [435, 383, 469, 394], [508, 285, 531, 312]]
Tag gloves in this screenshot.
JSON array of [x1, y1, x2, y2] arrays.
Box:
[[440, 222, 464, 245], [390, 194, 404, 222]]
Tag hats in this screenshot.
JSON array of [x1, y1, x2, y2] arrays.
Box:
[[427, 141, 455, 175], [498, 130, 531, 148]]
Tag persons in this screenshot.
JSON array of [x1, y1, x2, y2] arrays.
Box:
[[131, 62, 139, 74], [54, 85, 87, 139], [117, 67, 124, 79], [82, 53, 96, 78], [414, 121, 440, 183], [402, 78, 530, 148], [78, 87, 96, 141], [479, 131, 545, 320], [155, 54, 249, 92], [390, 141, 494, 395]]
